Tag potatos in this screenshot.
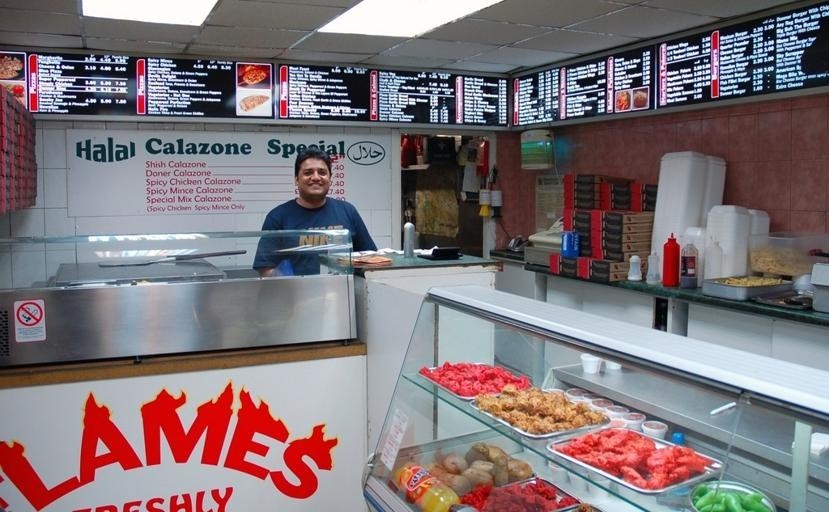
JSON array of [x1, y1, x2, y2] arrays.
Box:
[[427, 444, 531, 496]]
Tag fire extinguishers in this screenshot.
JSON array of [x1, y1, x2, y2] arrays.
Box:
[[476, 136, 489, 176]]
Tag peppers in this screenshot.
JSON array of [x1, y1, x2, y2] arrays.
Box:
[[693, 481, 769, 512]]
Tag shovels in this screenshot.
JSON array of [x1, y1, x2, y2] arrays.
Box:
[[99, 250, 246, 267]]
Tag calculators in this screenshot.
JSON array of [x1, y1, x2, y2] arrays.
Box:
[[417, 247, 460, 260]]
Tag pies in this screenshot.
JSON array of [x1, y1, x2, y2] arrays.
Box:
[[240, 96, 270, 112]]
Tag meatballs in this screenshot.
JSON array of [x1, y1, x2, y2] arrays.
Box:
[[476, 384, 603, 435]]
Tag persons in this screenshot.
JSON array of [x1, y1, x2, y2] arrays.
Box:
[[251, 144, 379, 278]]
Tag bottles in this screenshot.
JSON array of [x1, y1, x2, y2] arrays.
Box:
[[395, 462, 461, 512], [661, 232, 680, 287], [704, 241, 723, 280], [626, 254, 643, 281], [646, 252, 661, 285], [403, 216, 416, 258], [679, 242, 698, 289]]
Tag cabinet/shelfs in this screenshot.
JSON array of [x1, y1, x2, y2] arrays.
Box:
[[362, 284, 828, 511]]
